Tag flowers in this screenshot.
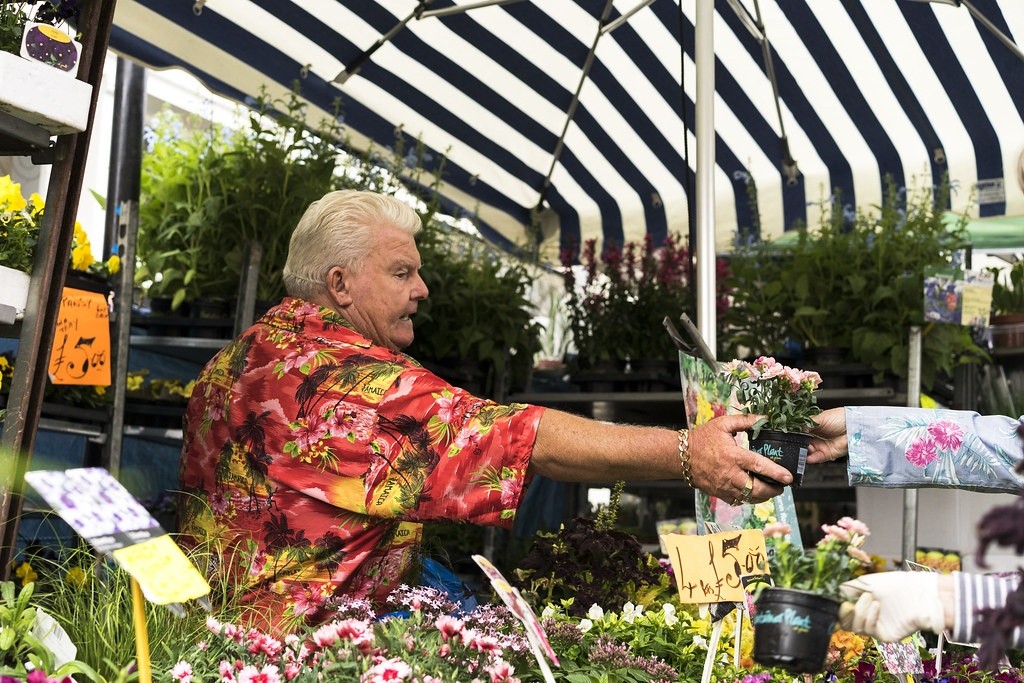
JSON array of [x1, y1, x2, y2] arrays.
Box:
[[718, 356, 827, 442], [0, 481, 1024, 683], [0, 173, 123, 277]]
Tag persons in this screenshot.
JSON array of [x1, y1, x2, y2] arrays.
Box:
[[172, 191, 794, 634], [800, 402, 1023, 645]]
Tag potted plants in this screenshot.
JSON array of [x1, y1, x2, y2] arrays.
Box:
[[533, 171, 1024, 392]]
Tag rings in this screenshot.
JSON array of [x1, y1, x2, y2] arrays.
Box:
[[728, 475, 754, 507]]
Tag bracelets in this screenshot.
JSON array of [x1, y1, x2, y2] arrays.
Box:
[[678, 429, 696, 491]]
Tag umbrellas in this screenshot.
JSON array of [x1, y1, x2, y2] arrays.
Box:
[[10, 0, 1023, 523]]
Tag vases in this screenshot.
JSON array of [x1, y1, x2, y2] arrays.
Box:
[[730, 427, 816, 489], [753, 584, 841, 675]]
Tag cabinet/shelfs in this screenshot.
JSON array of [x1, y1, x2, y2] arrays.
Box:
[[0, 1, 116, 598]]
